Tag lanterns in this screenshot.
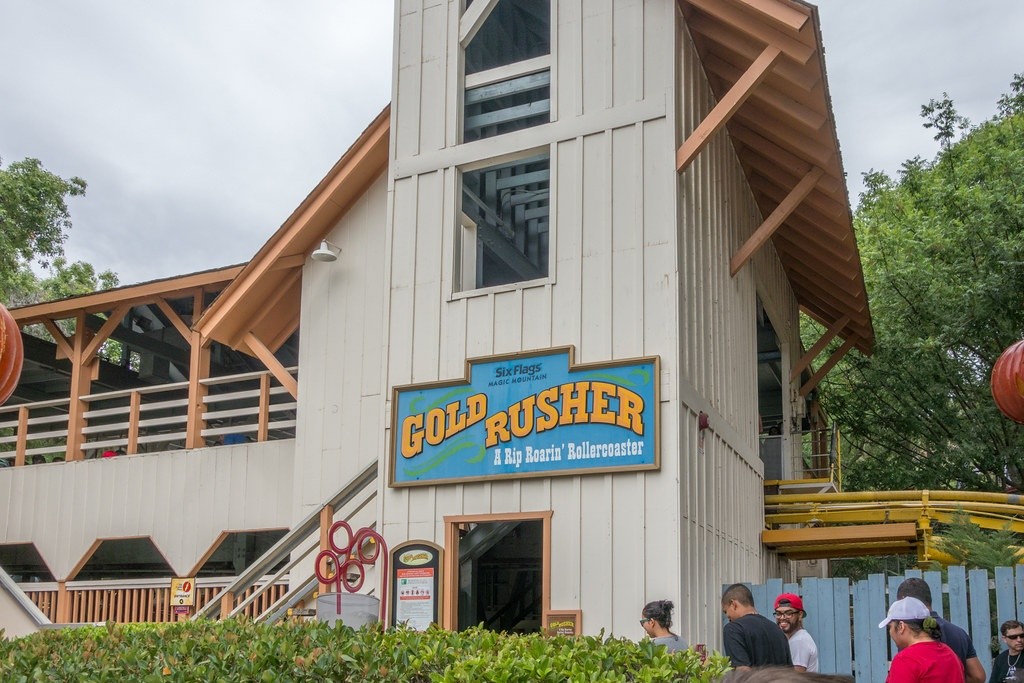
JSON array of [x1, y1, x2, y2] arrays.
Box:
[[991, 338, 1024, 425]]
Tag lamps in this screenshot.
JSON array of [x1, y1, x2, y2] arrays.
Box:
[[312, 239, 343, 262]]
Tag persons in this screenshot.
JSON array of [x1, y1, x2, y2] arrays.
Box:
[[885, 597, 966, 683], [218, 433, 251, 446], [897, 578, 986, 683], [772, 593, 819, 674], [989, 620, 1024, 683], [721, 584, 794, 671], [640, 600, 691, 654]]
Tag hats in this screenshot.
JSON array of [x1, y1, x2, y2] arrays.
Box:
[[878, 596, 930, 629], [774, 593, 807, 618]]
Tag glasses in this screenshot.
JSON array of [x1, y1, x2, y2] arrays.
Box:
[[1005, 633, 1024, 640], [772, 610, 800, 619], [639, 618, 650, 627]]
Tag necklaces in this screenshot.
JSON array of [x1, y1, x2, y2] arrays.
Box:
[[1007, 652, 1022, 668]]
